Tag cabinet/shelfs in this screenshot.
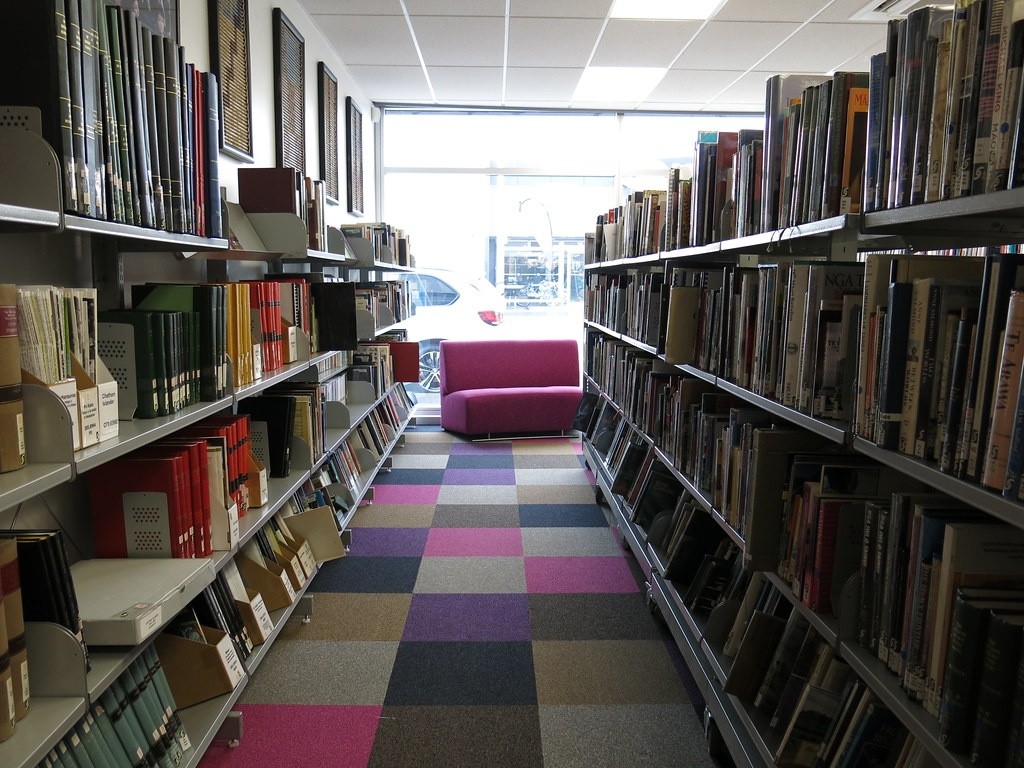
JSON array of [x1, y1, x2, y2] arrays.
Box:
[[0, 101, 415, 768], [575, 1, 1024, 768]]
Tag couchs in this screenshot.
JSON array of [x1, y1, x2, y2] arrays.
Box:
[[439, 337, 583, 443]]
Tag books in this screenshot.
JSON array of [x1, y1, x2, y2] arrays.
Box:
[[572, 0, 1024, 768], [0, 0, 417, 768]]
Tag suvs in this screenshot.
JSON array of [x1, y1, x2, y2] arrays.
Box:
[[382, 264, 508, 394]]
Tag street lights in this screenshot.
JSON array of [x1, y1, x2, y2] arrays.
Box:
[[517, 195, 554, 294]]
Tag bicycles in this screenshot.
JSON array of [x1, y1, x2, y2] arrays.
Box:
[[515, 277, 560, 309]]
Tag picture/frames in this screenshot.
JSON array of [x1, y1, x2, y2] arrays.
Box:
[[345, 95, 365, 216], [209, 0, 255, 162], [317, 61, 340, 204], [273, 7, 306, 177]]
